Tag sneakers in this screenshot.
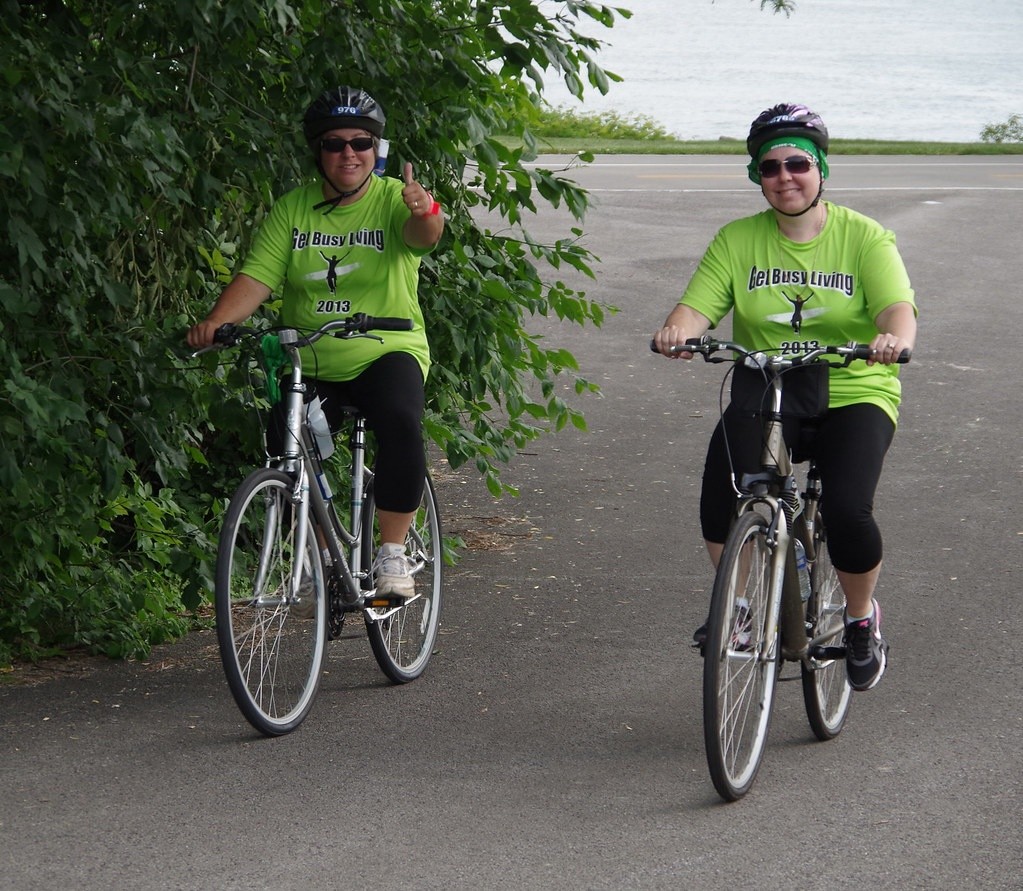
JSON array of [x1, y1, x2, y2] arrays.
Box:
[[844, 597, 888, 690], [693, 605, 751, 646], [294, 567, 327, 615], [375, 541, 416, 597]]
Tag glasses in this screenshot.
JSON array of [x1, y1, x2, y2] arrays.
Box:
[[760, 158, 816, 177], [316, 137, 373, 154]]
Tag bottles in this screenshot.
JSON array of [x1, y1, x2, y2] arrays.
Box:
[[789, 537, 812, 602], [301, 382, 334, 460]]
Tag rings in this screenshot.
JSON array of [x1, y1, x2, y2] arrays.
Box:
[[414, 201, 418, 207], [886, 344, 895, 348]]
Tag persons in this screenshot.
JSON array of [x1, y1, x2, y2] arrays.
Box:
[[188, 84, 445, 598], [655, 104, 919, 692]]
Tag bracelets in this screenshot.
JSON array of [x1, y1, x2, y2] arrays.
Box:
[[422, 192, 439, 217]]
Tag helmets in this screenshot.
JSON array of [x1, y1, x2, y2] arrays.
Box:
[[302, 86, 385, 136], [745, 106, 829, 154]]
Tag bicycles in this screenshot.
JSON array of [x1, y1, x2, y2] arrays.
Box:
[[651, 335, 912, 802], [187, 312, 445, 738]]
